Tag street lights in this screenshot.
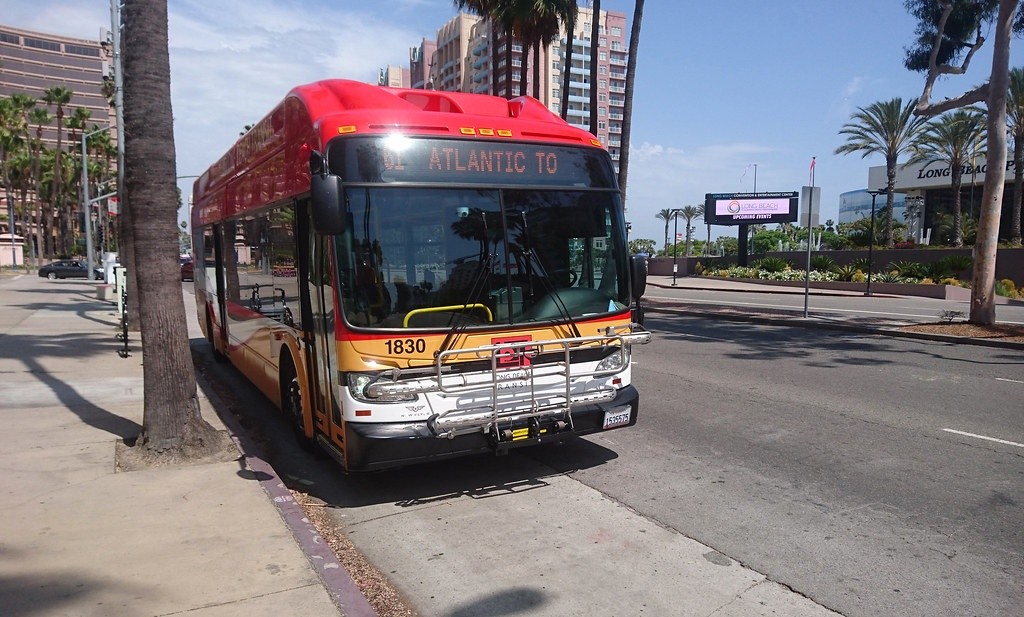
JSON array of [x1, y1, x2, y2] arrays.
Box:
[[670, 209, 681, 286], [624, 222, 633, 241], [864, 189, 879, 295]]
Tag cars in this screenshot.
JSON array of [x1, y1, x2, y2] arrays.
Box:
[[39, 259, 100, 280], [180, 253, 194, 282]]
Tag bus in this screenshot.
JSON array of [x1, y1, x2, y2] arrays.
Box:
[[190, 78, 651, 480]]
